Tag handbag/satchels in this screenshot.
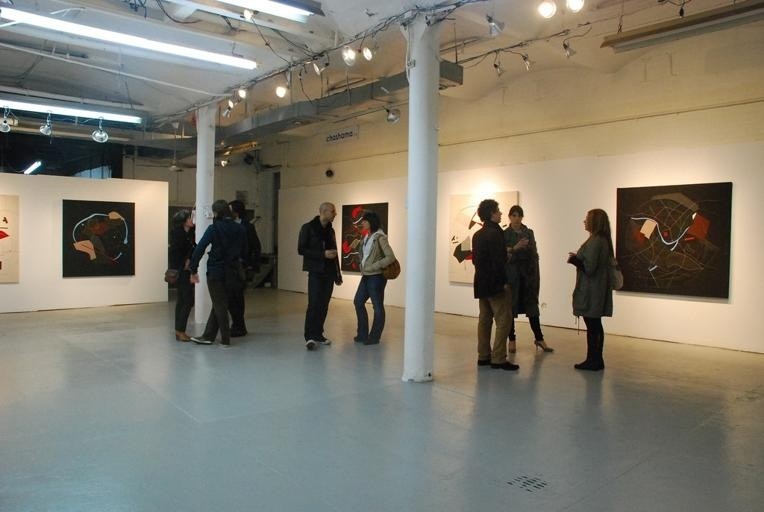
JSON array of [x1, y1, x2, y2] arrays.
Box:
[[165, 268, 181, 283], [382, 258, 400, 279], [607, 256, 624, 290]]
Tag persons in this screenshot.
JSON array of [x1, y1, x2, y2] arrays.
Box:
[[296, 201, 343, 349], [228, 200, 261, 336], [503, 204, 554, 354], [471, 198, 520, 370], [566, 209, 615, 371], [189, 200, 250, 345], [352, 210, 397, 346], [170, 208, 198, 342]]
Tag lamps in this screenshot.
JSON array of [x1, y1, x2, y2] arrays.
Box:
[[220, 38, 384, 119], [219, 1, 327, 24], [478, 2, 764, 76], [377, 98, 402, 125], [0, 3, 250, 72], [0, 87, 158, 143]]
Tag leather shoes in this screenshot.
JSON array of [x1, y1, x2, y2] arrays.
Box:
[[491, 361, 519, 370], [478, 359, 491, 366]]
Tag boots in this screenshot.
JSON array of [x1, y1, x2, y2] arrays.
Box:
[[574, 352, 604, 371]]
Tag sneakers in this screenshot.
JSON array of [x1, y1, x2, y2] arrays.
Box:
[[230, 328, 247, 337], [364, 338, 379, 345], [217, 341, 231, 348], [305, 338, 316, 351], [190, 337, 212, 345], [315, 335, 331, 345], [354, 336, 360, 342]]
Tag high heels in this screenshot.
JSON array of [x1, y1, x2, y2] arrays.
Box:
[[534, 339, 554, 352], [175, 330, 190, 341], [509, 342, 516, 353]]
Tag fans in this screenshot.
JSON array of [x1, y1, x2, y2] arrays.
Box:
[[136, 116, 201, 173]]
